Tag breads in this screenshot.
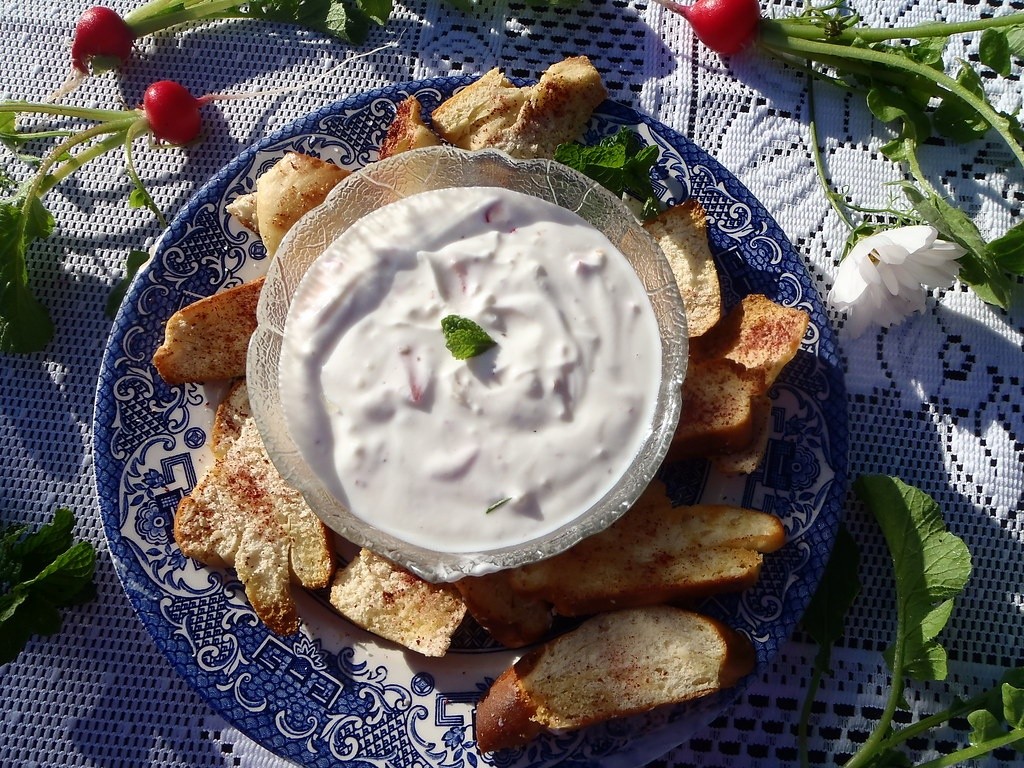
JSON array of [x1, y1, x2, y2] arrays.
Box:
[[154, 51, 811, 754]]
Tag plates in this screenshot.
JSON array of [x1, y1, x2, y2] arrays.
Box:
[[93, 76, 849, 768]]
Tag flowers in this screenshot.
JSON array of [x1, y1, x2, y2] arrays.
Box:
[[804, 0, 968, 336]]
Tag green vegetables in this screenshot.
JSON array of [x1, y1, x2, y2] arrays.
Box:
[[438, 314, 497, 360]]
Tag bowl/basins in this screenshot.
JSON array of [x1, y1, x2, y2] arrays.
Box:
[[246, 146, 690, 583]]
